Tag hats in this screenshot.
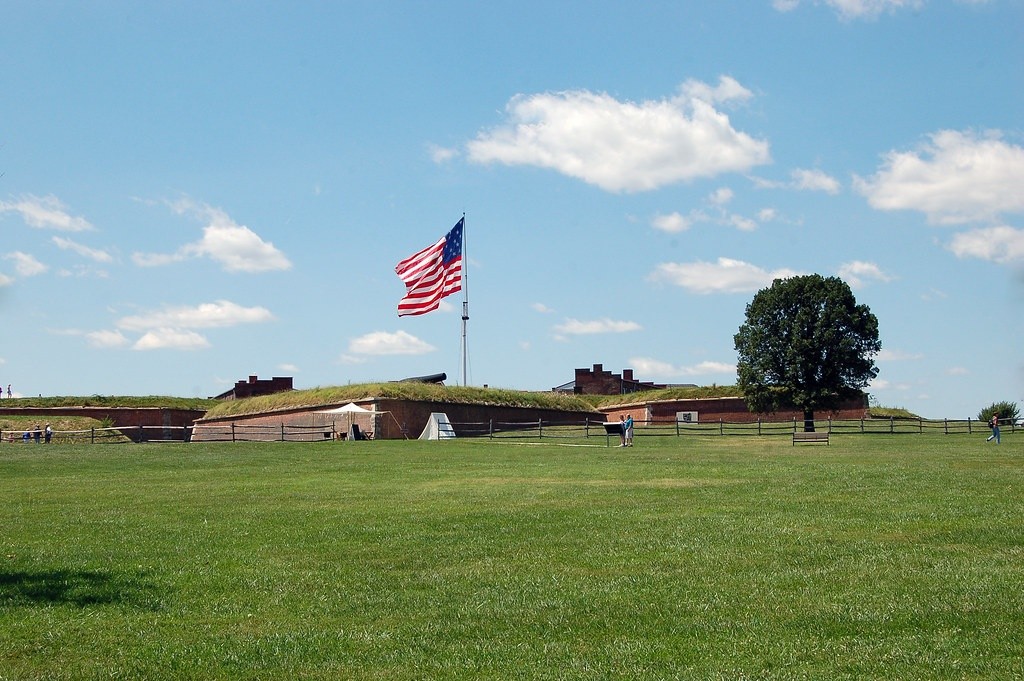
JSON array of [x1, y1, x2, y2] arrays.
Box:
[[46, 423, 50, 426]]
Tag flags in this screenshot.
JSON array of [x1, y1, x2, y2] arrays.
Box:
[[395, 216, 464, 316]]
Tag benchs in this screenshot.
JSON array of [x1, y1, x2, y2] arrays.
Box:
[[792, 432, 830, 447]]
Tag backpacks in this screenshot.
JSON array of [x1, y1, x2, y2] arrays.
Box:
[[988, 417, 996, 428]]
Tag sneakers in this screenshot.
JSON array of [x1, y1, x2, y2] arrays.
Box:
[[986, 439, 988, 443], [625, 443, 633, 447], [619, 444, 625, 447]]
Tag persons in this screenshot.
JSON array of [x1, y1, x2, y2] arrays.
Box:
[[624, 414, 633, 447], [0, 387, 3, 398], [985, 412, 1001, 444], [618, 414, 626, 447], [8, 432, 14, 443], [34, 425, 43, 444], [7, 384, 13, 398], [45, 423, 52, 443], [22, 427, 33, 443]]
